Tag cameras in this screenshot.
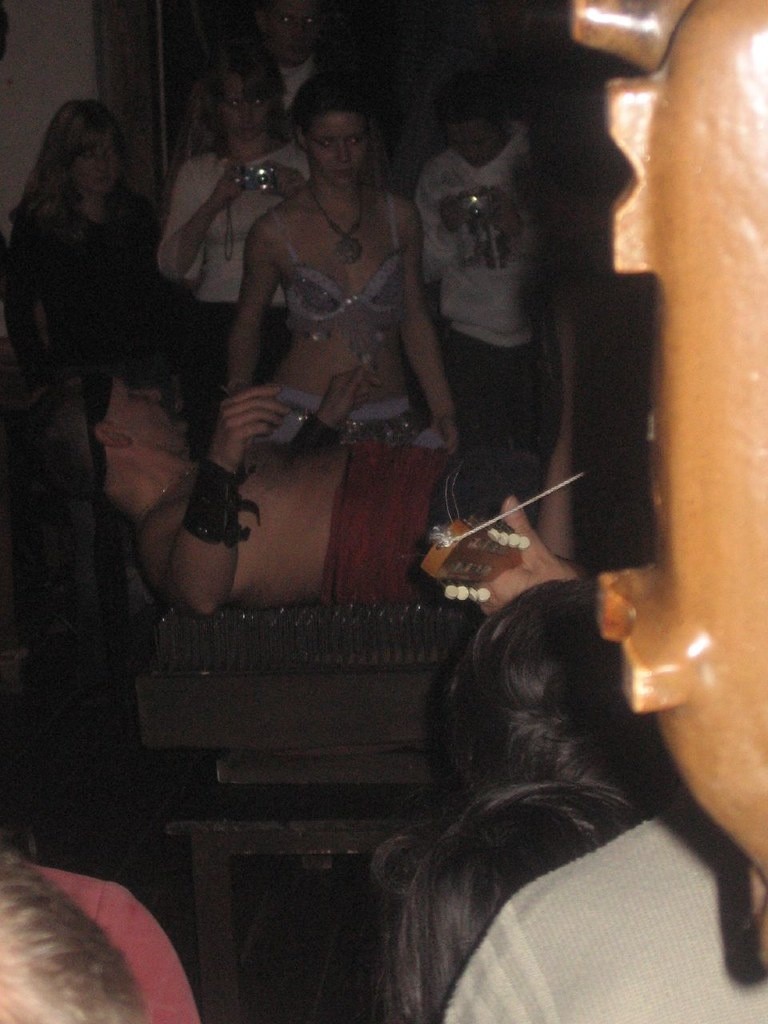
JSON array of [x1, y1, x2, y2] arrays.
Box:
[[457, 192, 485, 217], [232, 165, 277, 192]]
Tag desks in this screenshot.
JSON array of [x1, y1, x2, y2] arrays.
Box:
[[164, 784, 441, 1024]]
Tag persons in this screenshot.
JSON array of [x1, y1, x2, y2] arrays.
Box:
[[0, 0, 767, 1023]]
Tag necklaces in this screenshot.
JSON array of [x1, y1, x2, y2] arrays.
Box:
[[308, 185, 364, 265], [133, 461, 202, 520]]
